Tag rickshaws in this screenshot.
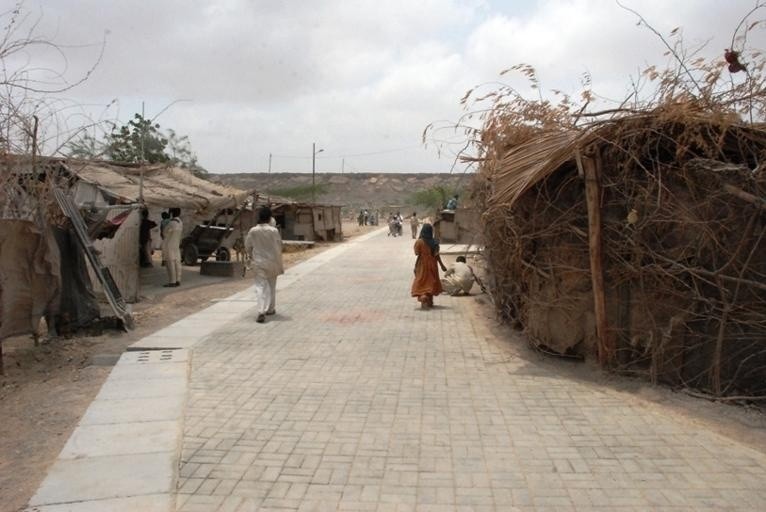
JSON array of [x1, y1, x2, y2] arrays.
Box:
[[180, 201, 249, 266]]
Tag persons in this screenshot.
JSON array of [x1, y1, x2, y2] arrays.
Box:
[[163, 207, 183, 288], [385, 212, 404, 237], [446, 195, 459, 209], [439, 256, 486, 296], [414, 224, 447, 306], [140, 210, 156, 268], [160, 212, 168, 266], [410, 213, 421, 240], [359, 208, 380, 225], [245, 206, 284, 322]]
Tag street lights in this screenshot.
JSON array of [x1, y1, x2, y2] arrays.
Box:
[[313, 143, 325, 202]]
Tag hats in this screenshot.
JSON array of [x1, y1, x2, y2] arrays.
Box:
[[260, 206, 272, 218]]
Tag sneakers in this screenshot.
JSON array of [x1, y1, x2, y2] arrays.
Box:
[[255, 308, 276, 323]]
